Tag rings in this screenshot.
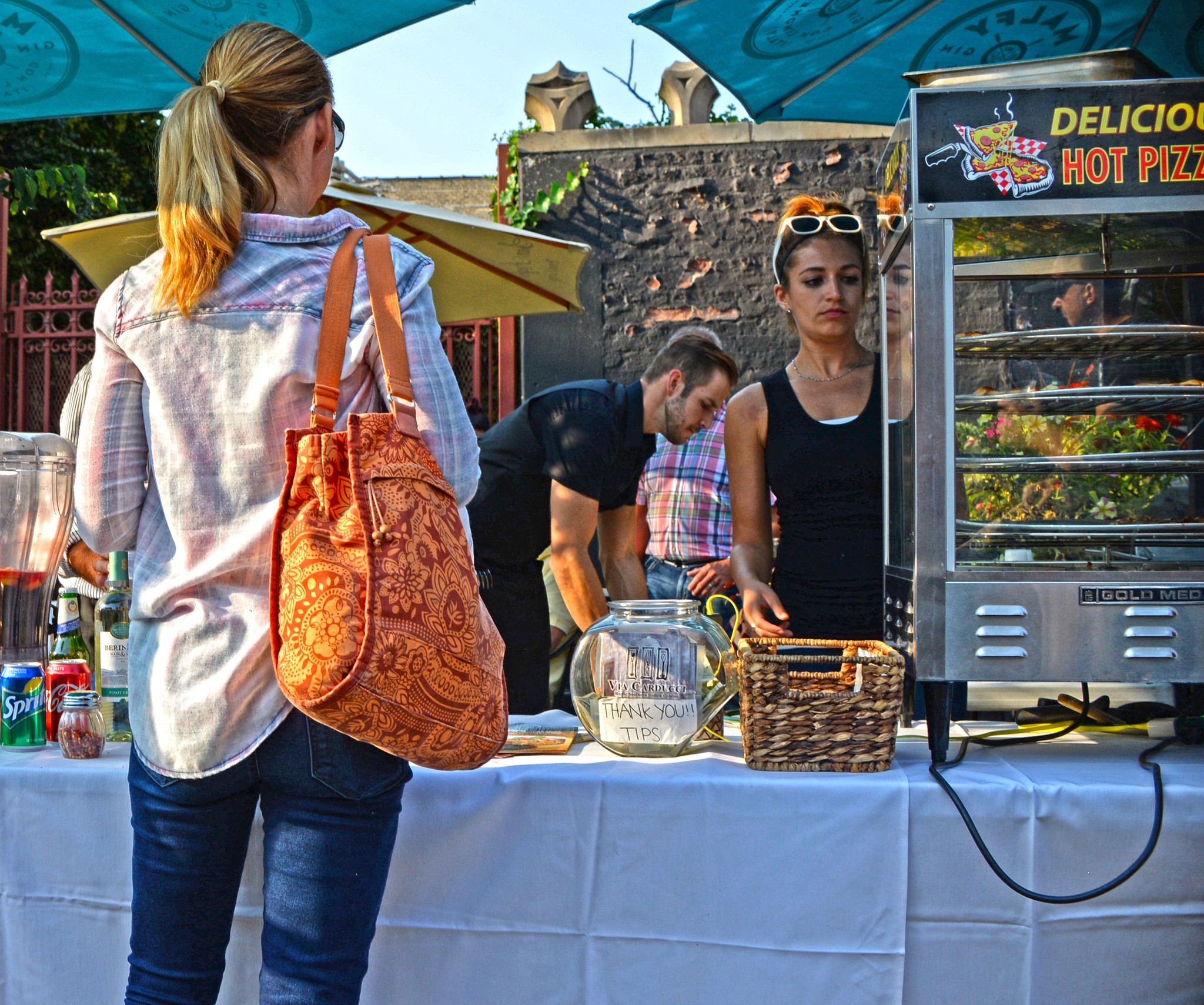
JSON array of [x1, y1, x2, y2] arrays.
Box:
[[708, 583, 712, 588]]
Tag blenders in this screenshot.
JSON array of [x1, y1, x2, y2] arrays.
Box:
[[0, 432, 76, 672]]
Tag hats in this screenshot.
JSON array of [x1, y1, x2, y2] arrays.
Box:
[[1024, 243, 1127, 297]]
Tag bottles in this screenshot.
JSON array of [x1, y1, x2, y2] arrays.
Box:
[[57, 690, 106, 759], [48, 588, 90, 666], [94, 551, 132, 743]]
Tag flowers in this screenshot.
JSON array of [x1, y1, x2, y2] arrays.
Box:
[[955, 360, 1188, 521]]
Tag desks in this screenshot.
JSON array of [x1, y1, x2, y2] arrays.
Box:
[[0, 720, 1204, 1005]]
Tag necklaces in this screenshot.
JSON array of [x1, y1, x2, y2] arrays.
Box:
[[790, 355, 864, 379]]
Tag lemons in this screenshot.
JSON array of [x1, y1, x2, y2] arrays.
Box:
[[1, 577, 41, 592]]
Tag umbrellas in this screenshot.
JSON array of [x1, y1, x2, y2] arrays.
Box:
[[0, 0, 1204, 334]]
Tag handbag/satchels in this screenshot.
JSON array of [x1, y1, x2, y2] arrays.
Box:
[[270, 230, 507, 771]]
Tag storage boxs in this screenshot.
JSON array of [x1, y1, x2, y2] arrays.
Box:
[[737, 638, 905, 773]]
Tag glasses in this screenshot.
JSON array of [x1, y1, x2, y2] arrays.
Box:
[[772, 214, 867, 284], [878, 214, 907, 233], [330, 110, 345, 155]]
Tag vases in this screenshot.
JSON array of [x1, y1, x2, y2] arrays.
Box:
[[1004, 549, 1034, 562]]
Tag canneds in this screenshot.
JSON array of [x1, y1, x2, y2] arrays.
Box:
[[0, 662, 46, 752], [44, 658, 92, 747]]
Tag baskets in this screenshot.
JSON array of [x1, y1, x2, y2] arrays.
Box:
[[736, 637, 905, 774]]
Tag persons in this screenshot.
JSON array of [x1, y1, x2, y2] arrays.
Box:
[[454, 193, 907, 716], [989, 241, 1175, 482], [876, 216, 972, 585], [59, 25, 486, 1005]]
